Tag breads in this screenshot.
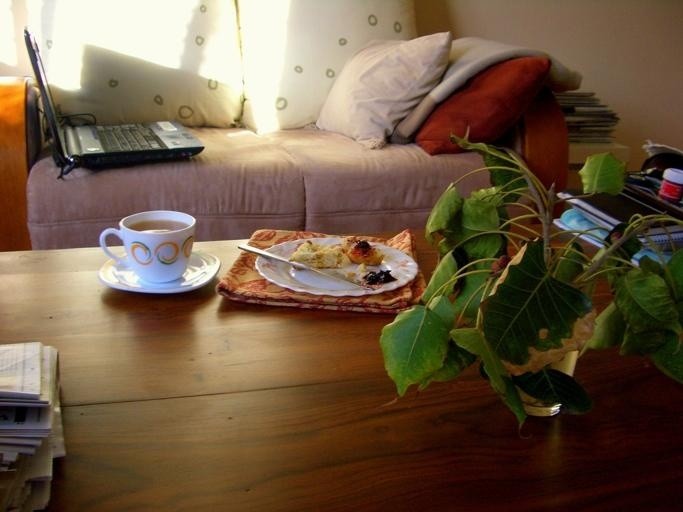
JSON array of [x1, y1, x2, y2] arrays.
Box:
[[345, 236, 386, 266]]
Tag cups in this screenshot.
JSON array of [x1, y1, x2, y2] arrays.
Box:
[[97, 209, 195, 285]]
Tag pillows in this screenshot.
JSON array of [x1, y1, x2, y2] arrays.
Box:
[[414, 55, 552, 156], [236, 0, 417, 134], [315, 31, 452, 149], [25, 0, 242, 128]]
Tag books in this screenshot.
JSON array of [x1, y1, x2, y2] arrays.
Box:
[[551, 171, 683, 268], [554, 91, 630, 166]]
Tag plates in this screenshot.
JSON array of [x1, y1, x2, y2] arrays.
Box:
[[255, 237, 418, 298], [97, 250, 220, 294]]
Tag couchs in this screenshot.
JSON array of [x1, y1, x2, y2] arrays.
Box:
[[0, 75, 570, 250]]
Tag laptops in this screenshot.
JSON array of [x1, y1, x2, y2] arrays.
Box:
[[24, 25, 204, 179]]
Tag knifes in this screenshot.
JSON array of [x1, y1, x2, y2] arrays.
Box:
[[235, 243, 374, 291]]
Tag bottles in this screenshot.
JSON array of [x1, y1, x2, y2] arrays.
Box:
[[659, 166, 683, 205]]
